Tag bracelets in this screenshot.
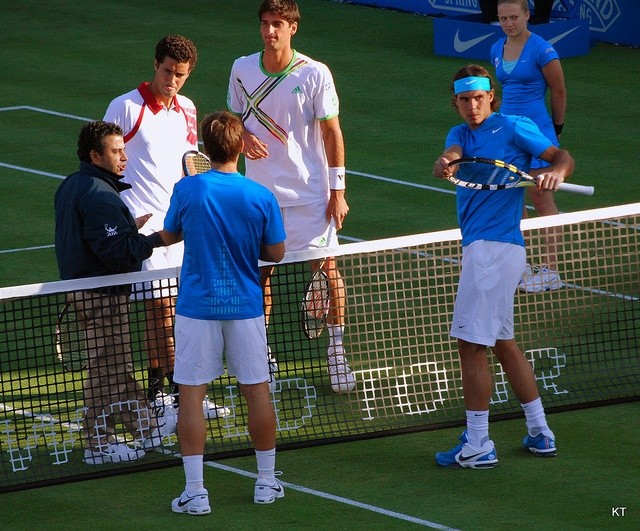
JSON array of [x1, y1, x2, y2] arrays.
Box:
[[328, 167, 346, 192], [553, 122, 564, 136]]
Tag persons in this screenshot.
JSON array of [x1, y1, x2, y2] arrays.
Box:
[[54, 122, 176, 465], [489, 0, 568, 296], [161, 110, 286, 517], [226, 1, 356, 396], [435, 63, 576, 472], [103, 71, 231, 421]]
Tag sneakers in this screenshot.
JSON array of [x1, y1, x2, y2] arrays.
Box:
[[202, 397, 231, 419], [519, 266, 562, 293], [146, 407, 177, 447], [435, 432, 499, 469], [171, 487, 212, 516], [151, 391, 176, 406], [523, 429, 558, 458], [84, 441, 145, 464], [254, 471, 285, 505], [327, 347, 355, 394]]
